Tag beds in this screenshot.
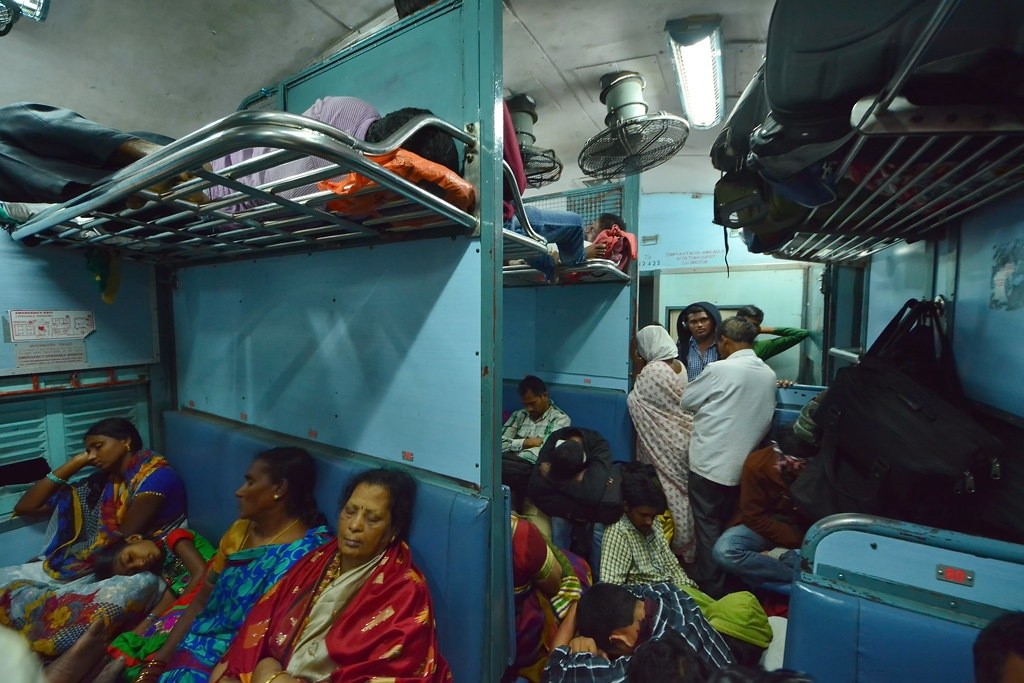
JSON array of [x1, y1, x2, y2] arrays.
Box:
[[710, 0, 1024, 266], [501, 180, 630, 286], [10, 109, 483, 271]]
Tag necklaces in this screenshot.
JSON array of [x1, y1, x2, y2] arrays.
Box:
[[240, 515, 301, 549]]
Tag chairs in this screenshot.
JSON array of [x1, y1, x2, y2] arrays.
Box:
[[768, 384, 829, 445], [783, 514, 1024, 683]]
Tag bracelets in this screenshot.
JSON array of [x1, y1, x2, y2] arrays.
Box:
[[266, 670, 288, 683], [136, 659, 167, 683], [147, 613, 158, 623], [47, 472, 68, 484]]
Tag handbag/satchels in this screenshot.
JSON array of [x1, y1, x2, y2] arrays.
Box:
[[788, 296, 1024, 547], [712, 0, 1024, 256]]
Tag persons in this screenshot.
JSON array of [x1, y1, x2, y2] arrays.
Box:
[[134, 446, 337, 683], [94, 527, 217, 683], [973, 612, 1024, 682], [42, 618, 108, 683], [503, 97, 626, 286], [500, 302, 817, 683], [210, 467, 452, 683], [0, 96, 460, 234], [93, 656, 124, 683], [14, 416, 189, 582]]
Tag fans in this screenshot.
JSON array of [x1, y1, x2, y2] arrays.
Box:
[[507, 94, 565, 190], [577, 71, 692, 180]]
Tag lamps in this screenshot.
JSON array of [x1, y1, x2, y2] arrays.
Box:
[[663, 13, 726, 130]]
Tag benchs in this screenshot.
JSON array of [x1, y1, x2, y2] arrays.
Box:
[[159, 407, 490, 683], [504, 377, 632, 467]]
